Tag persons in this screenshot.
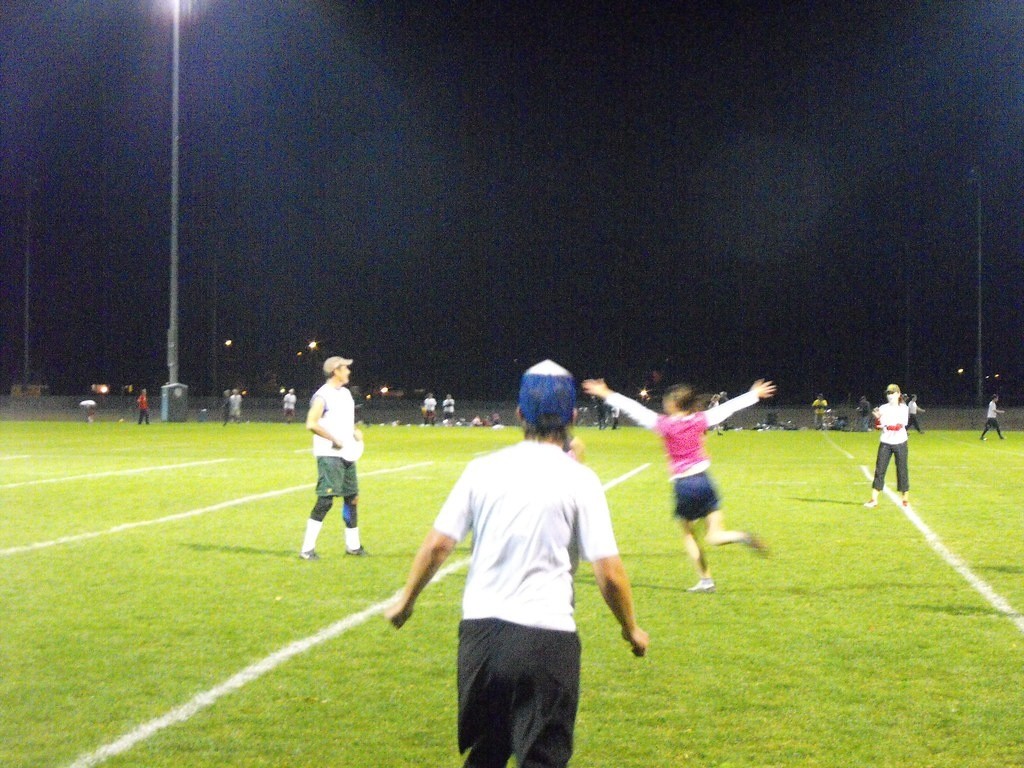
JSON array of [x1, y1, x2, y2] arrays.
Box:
[[283, 388, 297, 424], [221, 389, 243, 426], [456, 417, 465, 426], [592, 394, 620, 430], [905, 395, 926, 434], [863, 384, 909, 509], [299, 356, 370, 560], [136, 388, 151, 425], [472, 410, 500, 426], [704, 392, 732, 437], [88, 404, 94, 423], [442, 393, 455, 427], [383, 359, 649, 768], [423, 392, 437, 427], [582, 376, 776, 593], [980, 394, 1007, 441], [811, 393, 870, 433]]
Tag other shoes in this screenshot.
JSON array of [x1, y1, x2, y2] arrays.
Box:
[[745, 532, 764, 551], [902, 494, 907, 506], [689, 578, 715, 593], [864, 499, 877, 507]]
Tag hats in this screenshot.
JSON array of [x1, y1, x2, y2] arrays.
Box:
[[323, 357, 355, 377], [885, 384, 900, 394], [518, 359, 575, 431]]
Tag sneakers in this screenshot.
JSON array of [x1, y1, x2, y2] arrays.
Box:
[[346, 546, 369, 557], [300, 550, 322, 562]]
